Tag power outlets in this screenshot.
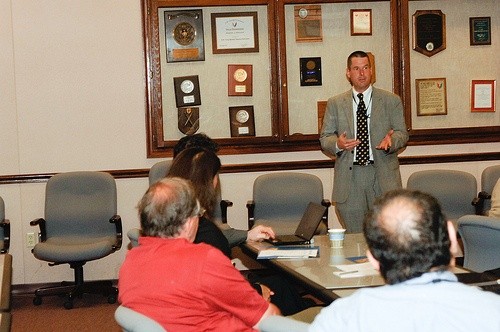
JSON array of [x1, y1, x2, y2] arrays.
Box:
[[26, 233, 36, 248]]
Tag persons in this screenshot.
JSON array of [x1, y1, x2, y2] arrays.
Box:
[[319, 50, 409, 234], [173, 133, 275, 246], [118, 177, 324, 330], [308, 189, 500, 332], [169, 148, 306, 315]]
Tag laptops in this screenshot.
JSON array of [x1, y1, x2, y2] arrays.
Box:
[[263, 201, 327, 246]]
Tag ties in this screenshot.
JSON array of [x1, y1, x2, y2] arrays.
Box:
[[356, 94, 369, 166]]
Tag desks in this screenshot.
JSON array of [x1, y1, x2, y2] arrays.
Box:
[[241, 233, 500, 306]]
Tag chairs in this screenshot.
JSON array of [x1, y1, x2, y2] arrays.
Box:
[[247, 172, 331, 235], [115, 303, 166, 332], [30, 172, 122, 310], [149, 160, 233, 224], [407, 165, 500, 277]]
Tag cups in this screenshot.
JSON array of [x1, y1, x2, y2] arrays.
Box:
[[329, 248, 344, 265], [328, 229, 346, 247]]
